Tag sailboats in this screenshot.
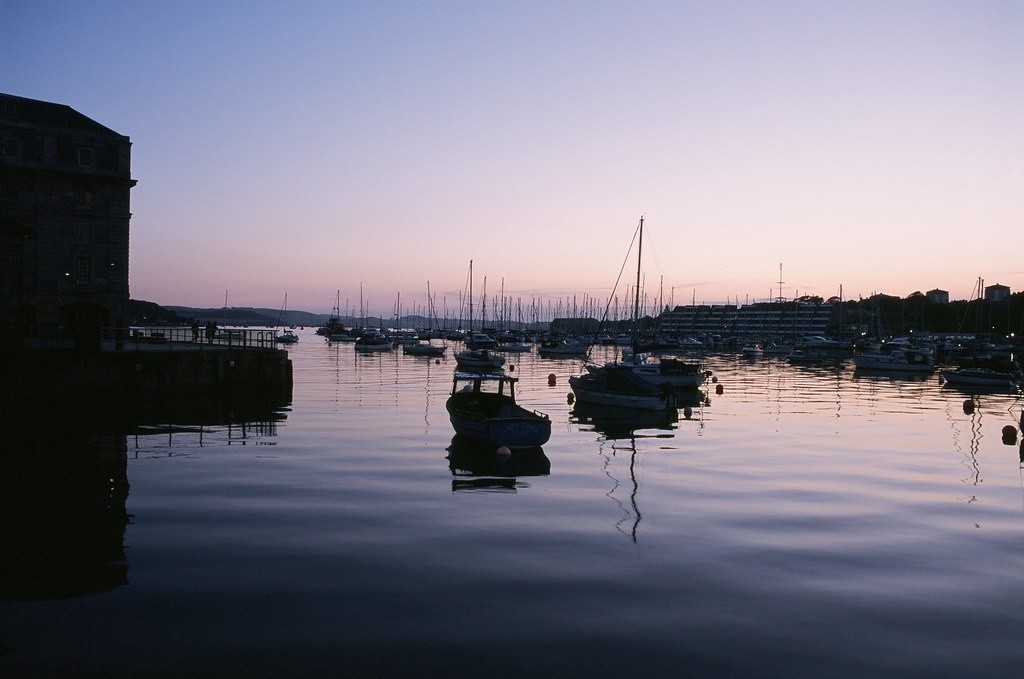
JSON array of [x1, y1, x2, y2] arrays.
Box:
[[212, 289, 246, 341], [272, 293, 300, 344], [311, 209, 1024, 390]]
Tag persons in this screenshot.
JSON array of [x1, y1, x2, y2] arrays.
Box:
[[205, 321, 218, 342], [192, 320, 201, 342]]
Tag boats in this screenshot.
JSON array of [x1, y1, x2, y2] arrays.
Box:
[[444, 368, 555, 450], [444, 435, 557, 479]]
